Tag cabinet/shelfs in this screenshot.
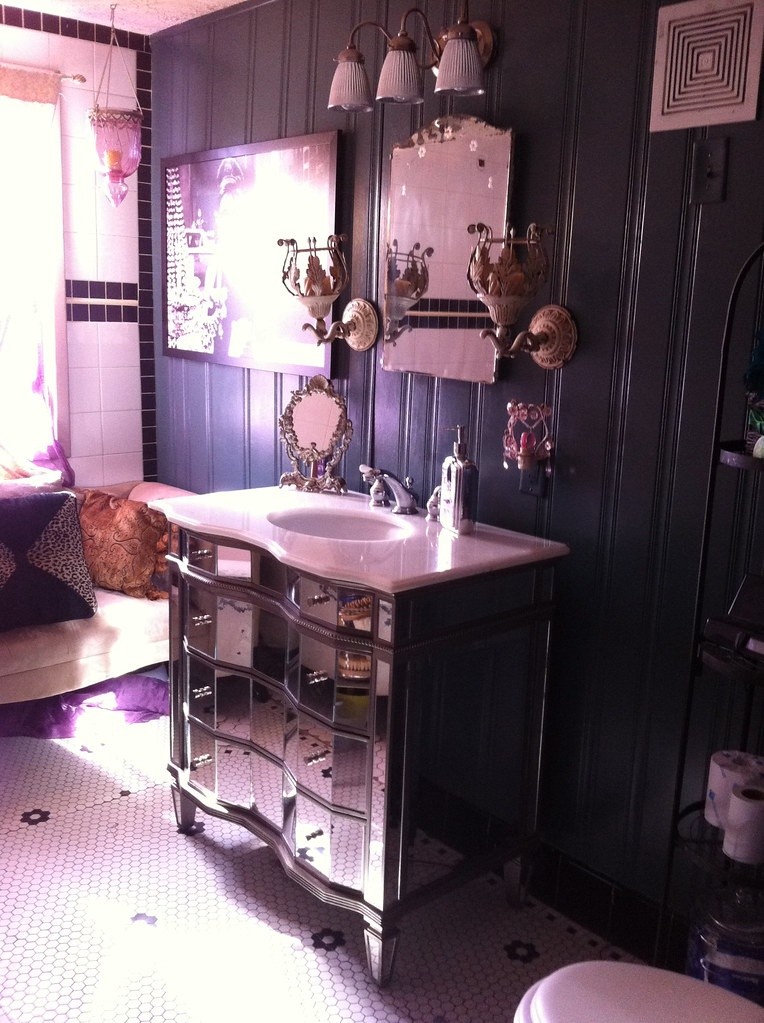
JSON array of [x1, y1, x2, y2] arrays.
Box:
[[646, 224, 764, 982]]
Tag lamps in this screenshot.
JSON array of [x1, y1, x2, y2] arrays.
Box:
[[384, 234, 436, 346], [326, 0, 495, 114], [87, 2, 145, 209], [276, 233, 378, 352], [464, 221, 579, 369], [503, 399, 555, 478]]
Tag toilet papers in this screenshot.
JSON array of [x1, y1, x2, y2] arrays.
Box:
[[703, 749, 764, 866]]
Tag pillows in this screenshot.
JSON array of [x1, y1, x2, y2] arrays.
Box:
[[72, 488, 168, 601], [0, 491, 99, 634], [146, 521, 181, 600]]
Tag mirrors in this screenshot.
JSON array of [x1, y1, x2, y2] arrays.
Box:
[[278, 373, 355, 496], [379, 112, 515, 389]]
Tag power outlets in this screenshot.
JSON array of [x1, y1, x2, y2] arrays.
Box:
[[517, 461, 547, 498]]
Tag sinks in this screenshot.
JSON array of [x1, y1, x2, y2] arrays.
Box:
[[267, 505, 417, 542]]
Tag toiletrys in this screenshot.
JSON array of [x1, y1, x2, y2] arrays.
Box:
[[437, 423, 479, 534]]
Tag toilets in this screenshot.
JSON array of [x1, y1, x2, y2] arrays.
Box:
[[513, 959, 764, 1021]]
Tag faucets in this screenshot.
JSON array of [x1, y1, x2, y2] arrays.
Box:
[[358, 463, 420, 514]]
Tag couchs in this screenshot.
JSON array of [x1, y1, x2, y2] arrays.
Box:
[[0, 479, 217, 707]]
